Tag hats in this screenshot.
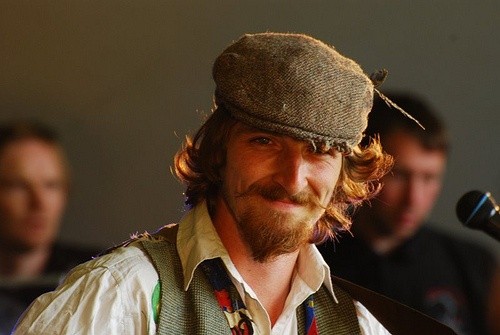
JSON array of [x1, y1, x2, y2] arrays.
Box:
[[213, 33, 374, 155]]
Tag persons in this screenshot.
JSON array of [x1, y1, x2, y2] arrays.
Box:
[[317, 90, 500, 334], [0, 117, 74, 283], [7, 31, 395, 335]]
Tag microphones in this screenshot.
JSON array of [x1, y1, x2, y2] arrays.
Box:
[[456, 190, 500, 242]]
